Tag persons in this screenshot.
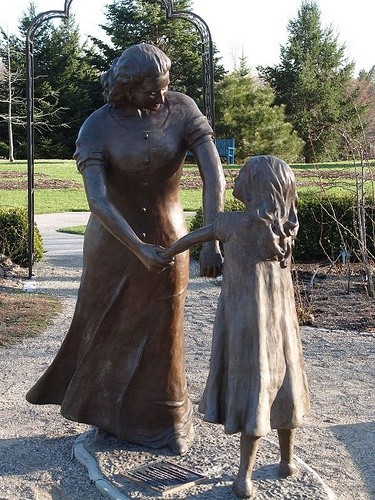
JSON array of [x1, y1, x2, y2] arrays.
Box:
[[157, 155, 311, 498], [25, 42, 225, 457]]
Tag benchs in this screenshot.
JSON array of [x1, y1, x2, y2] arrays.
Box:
[[187, 138, 236, 166]]
